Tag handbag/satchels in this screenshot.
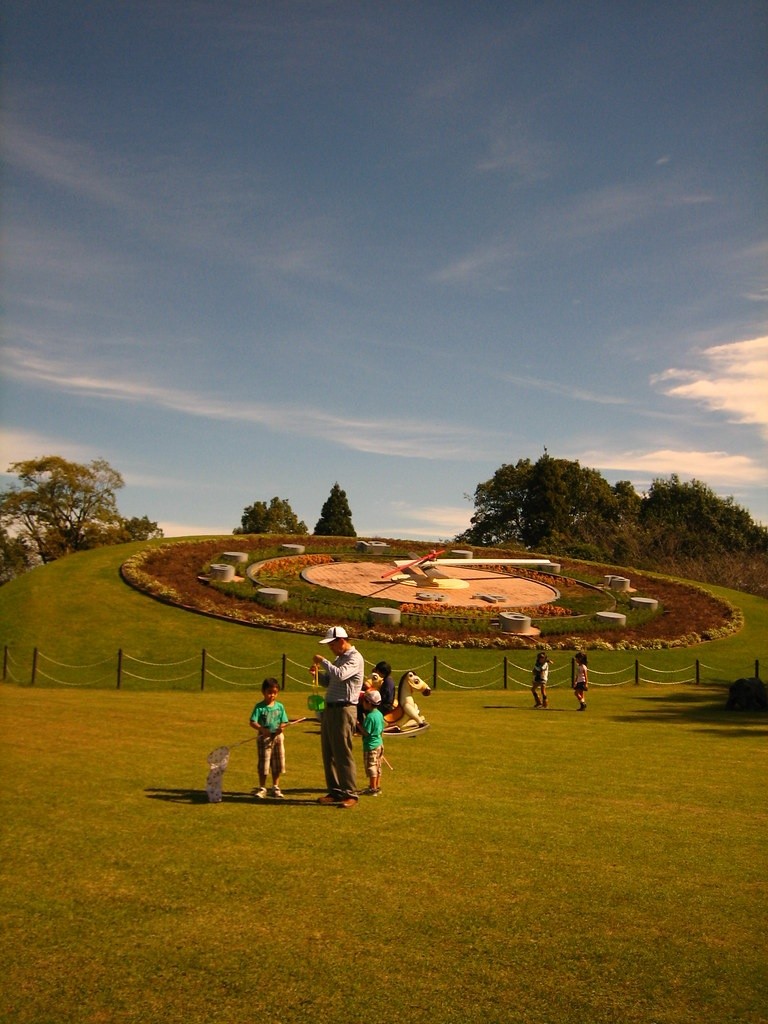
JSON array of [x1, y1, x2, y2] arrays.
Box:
[[307, 694, 324, 711]]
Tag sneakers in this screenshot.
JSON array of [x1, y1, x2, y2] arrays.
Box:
[[342, 796, 357, 807], [318, 796, 343, 804]]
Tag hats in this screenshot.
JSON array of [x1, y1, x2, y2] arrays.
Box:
[[365, 690, 382, 705], [318, 627, 348, 644]]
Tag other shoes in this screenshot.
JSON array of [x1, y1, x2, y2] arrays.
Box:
[[272, 787, 283, 797], [577, 703, 586, 711], [543, 699, 549, 708], [256, 787, 267, 798], [358, 786, 382, 796], [534, 702, 543, 708]]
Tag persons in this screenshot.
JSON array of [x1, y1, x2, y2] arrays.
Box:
[[530, 652, 553, 710], [309, 626, 365, 808], [250, 678, 289, 798], [572, 652, 589, 711], [353, 660, 396, 737], [356, 690, 384, 797]]
